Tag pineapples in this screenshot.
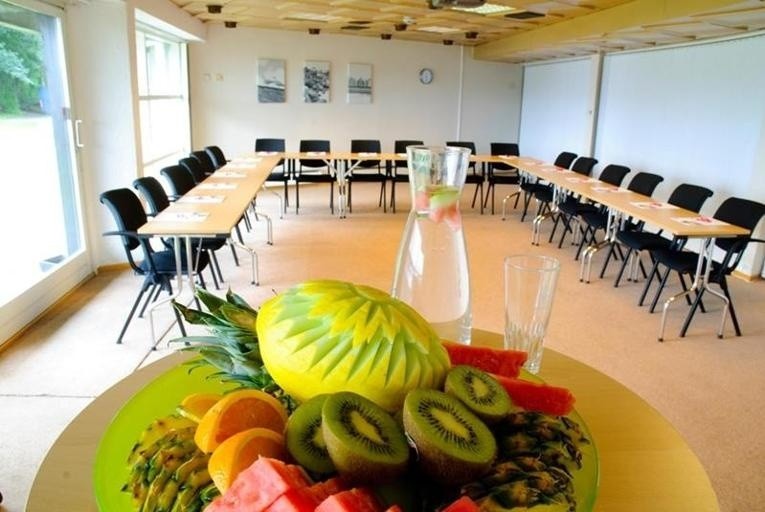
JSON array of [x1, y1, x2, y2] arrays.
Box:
[[121, 417, 221, 512], [473, 410, 588, 512]]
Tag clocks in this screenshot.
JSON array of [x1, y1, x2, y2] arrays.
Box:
[[418, 67, 434, 85]]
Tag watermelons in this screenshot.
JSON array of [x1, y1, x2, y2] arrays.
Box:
[[206, 456, 313, 512], [443, 340, 529, 377], [315, 487, 388, 512], [488, 373, 575, 416], [262, 478, 348, 512]]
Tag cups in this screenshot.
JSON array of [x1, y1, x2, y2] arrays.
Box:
[[501, 254, 561, 376]]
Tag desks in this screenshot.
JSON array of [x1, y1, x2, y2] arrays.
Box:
[[500, 156, 753, 343], [137, 155, 282, 351], [258, 153, 503, 219]]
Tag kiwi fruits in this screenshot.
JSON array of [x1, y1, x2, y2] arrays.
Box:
[[445, 364, 511, 428], [323, 392, 409, 489], [284, 393, 335, 474], [403, 388, 497, 484]]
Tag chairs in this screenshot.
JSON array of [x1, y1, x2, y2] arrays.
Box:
[[392, 139, 424, 214], [131, 175, 226, 291], [252, 137, 290, 216], [159, 163, 241, 273], [583, 171, 664, 287], [648, 196, 765, 344], [614, 183, 715, 307], [519, 152, 578, 223], [446, 141, 485, 216], [203, 145, 259, 228], [99, 187, 211, 347], [189, 150, 250, 238], [293, 139, 338, 216], [343, 139, 389, 214], [177, 155, 243, 253], [483, 142, 528, 215], [556, 163, 630, 261], [535, 154, 598, 249]]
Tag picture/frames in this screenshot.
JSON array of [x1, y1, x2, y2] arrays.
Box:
[[347, 61, 374, 103], [303, 60, 332, 104], [256, 57, 288, 103]]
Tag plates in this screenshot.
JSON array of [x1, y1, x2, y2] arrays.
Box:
[[90, 359, 600, 512]]
